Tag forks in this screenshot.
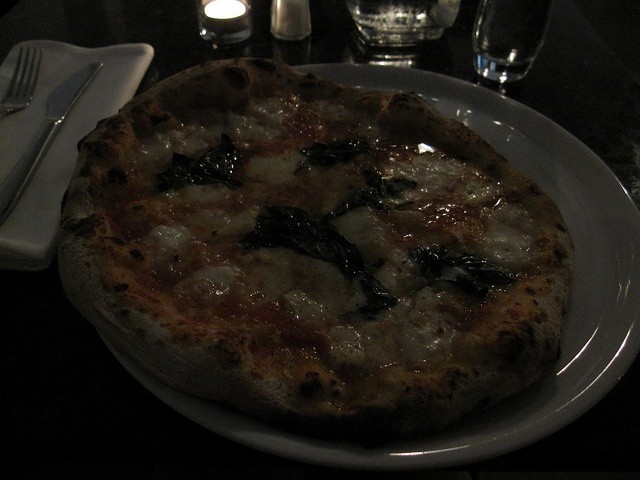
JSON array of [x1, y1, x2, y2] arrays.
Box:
[[0, 47, 46, 126]]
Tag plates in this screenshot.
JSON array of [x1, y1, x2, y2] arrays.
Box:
[[56, 63, 640, 472]]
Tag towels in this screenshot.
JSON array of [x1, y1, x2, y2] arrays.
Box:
[[0, 36, 159, 278]]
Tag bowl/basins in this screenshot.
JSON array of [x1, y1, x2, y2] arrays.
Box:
[[348, 0, 459, 55]]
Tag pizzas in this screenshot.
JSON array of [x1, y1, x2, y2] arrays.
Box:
[[55, 57, 575, 434]]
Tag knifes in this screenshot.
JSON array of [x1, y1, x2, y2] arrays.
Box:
[[0, 59, 103, 230]]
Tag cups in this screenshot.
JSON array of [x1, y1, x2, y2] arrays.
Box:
[[196, 0, 254, 42], [470, 0, 550, 83]]
